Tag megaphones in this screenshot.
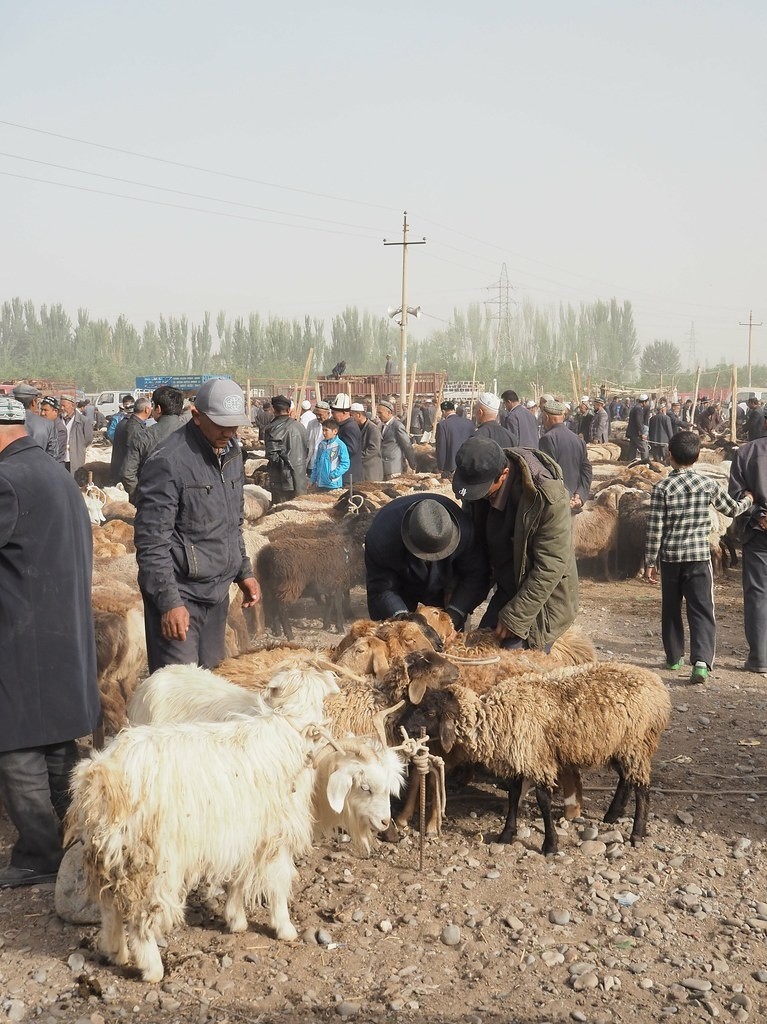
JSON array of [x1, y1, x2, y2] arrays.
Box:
[[388, 306, 402, 318], [406, 305, 421, 318]]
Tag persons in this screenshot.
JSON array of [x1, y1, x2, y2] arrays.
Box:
[[107, 394, 196, 482], [289, 392, 472, 485], [436, 401, 475, 477], [365, 493, 491, 631], [471, 393, 519, 448], [134, 378, 261, 676], [385, 354, 392, 374], [118, 386, 188, 507], [452, 437, 580, 655], [307, 418, 350, 492], [501, 390, 539, 450], [643, 433, 753, 684], [0, 397, 102, 887], [538, 401, 592, 514], [265, 395, 309, 505], [728, 401, 767, 672], [525, 394, 767, 462], [327, 361, 346, 381], [0, 381, 107, 479]]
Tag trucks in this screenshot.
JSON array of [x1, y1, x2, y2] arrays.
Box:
[[238, 384, 279, 404], [135, 374, 231, 402], [676, 388, 730, 407], [441, 380, 486, 414], [0, 378, 77, 400], [286, 371, 445, 422], [722, 387, 767, 428], [94, 388, 156, 420]]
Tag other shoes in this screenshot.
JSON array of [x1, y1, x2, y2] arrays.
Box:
[[666, 657, 684, 669], [690, 664, 711, 684], [744, 658, 767, 672], [0, 863, 65, 888]]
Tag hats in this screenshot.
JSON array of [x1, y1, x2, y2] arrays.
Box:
[[452, 437, 505, 500], [480, 392, 502, 412], [671, 402, 681, 406], [301, 400, 311, 410], [595, 398, 604, 404], [193, 376, 252, 426], [313, 402, 331, 414], [262, 402, 271, 410], [638, 393, 648, 402], [657, 402, 665, 410], [332, 393, 352, 411], [350, 402, 364, 412], [581, 396, 589, 402], [62, 394, 75, 403], [544, 401, 565, 415], [583, 401, 590, 409], [272, 395, 291, 408], [43, 396, 60, 410], [400, 499, 461, 560], [440, 402, 454, 410], [0, 398, 26, 425], [379, 399, 394, 412], [13, 384, 42, 398]]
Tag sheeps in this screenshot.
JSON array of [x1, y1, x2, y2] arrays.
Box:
[[570, 421, 738, 583], [73, 442, 598, 747], [61, 654, 373, 985], [395, 662, 672, 857], [123, 663, 408, 856]]
